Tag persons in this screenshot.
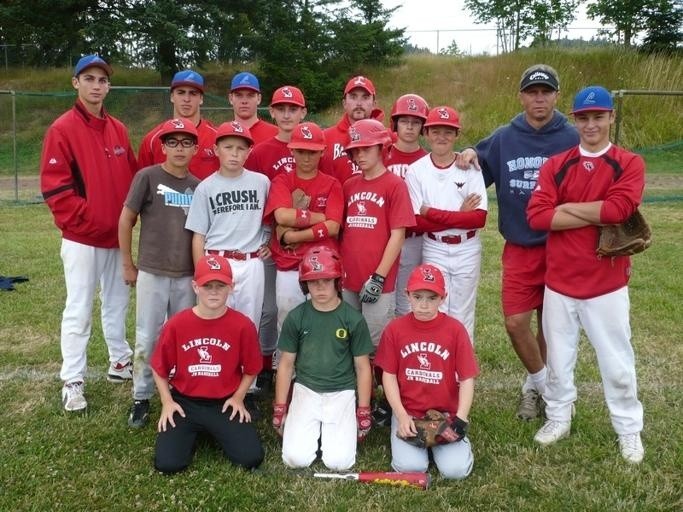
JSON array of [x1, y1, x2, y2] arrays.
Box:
[[524, 85, 644, 466], [273, 248, 375, 467], [151, 255, 265, 470], [116, 70, 488, 429], [455, 65, 577, 422], [378, 264, 480, 478], [40, 54, 138, 412]]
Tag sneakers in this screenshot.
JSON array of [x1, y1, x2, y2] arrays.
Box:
[[127, 399, 150, 429], [61, 381, 87, 412], [370, 402, 391, 430], [106, 360, 133, 384], [615, 432, 644, 465], [532, 420, 570, 446], [271, 351, 296, 384], [515, 389, 541, 420]]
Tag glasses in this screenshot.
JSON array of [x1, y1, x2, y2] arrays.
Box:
[[162, 136, 196, 148]]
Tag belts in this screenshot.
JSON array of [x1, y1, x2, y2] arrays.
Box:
[[207, 250, 258, 261], [427, 230, 474, 244], [404, 230, 423, 238]]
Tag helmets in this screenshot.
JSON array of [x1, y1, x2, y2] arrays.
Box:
[[340, 118, 393, 166], [390, 94, 429, 132], [298, 246, 344, 295]]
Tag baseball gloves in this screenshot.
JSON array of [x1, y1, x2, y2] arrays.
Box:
[[596, 208, 653, 257], [397, 409, 447, 448]]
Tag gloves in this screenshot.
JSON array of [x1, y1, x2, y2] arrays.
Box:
[[356, 406, 372, 442], [272, 404, 287, 438], [357, 273, 384, 304], [434, 415, 469, 445]]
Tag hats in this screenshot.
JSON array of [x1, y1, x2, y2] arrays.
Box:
[[286, 122, 325, 151], [214, 121, 255, 145], [567, 86, 613, 115], [73, 54, 113, 77], [343, 76, 374, 95], [170, 70, 305, 107], [158, 118, 198, 137], [519, 70, 558, 91], [406, 264, 444, 297], [194, 255, 232, 287], [423, 106, 459, 129]]
